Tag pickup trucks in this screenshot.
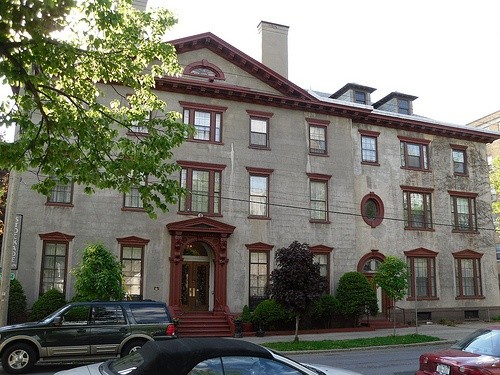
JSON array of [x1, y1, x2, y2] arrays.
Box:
[[0, 300, 178, 375]]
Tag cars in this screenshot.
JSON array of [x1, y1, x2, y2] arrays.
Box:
[[414, 325, 500, 375], [51, 337, 366, 375]]
